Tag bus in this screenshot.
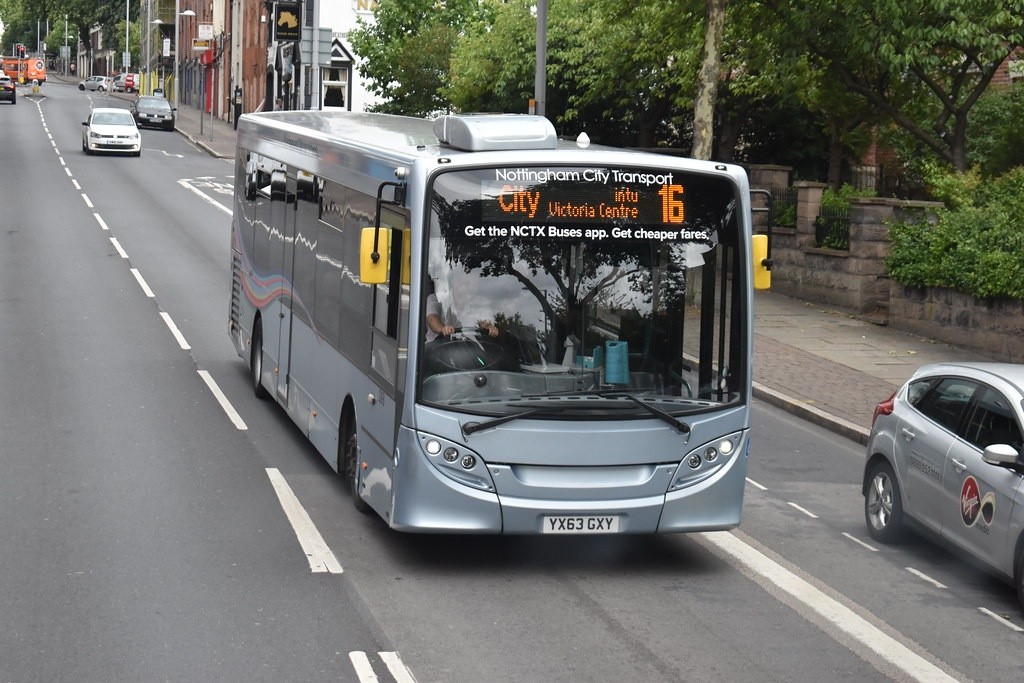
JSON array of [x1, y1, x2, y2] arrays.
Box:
[[228, 109, 774, 532]]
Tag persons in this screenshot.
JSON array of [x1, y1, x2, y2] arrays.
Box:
[[425, 270, 499, 345], [275, 98, 283, 112], [70, 63, 76, 75]]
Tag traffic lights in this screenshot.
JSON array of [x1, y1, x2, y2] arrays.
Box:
[[16, 44, 25, 59]]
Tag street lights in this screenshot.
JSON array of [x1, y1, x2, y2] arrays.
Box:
[[146, 20, 163, 95], [175, 10, 196, 119]]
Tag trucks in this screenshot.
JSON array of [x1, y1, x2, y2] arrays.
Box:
[[0, 56, 47, 86]]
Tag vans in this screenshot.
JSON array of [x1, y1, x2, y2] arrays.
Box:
[[113, 74, 139, 94]]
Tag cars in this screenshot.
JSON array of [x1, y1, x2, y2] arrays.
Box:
[[130, 96, 178, 132], [82, 107, 142, 156], [0, 76, 16, 105], [79, 76, 110, 92], [863, 362, 1024, 617]]
